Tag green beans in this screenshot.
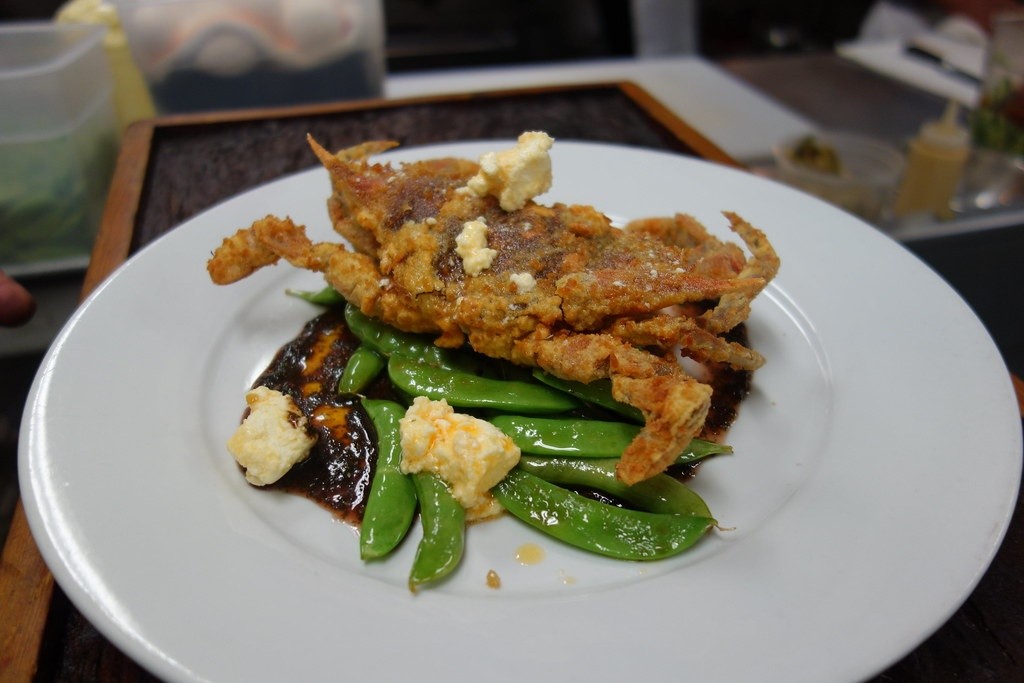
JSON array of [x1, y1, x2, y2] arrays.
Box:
[[285, 285, 733, 592]]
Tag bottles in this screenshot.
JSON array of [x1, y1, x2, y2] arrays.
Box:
[[891, 100, 970, 223]]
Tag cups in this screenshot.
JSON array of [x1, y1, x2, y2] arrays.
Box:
[[771, 134, 906, 225]]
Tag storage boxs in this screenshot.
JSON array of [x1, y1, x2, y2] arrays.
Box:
[[0, 0, 389, 264]]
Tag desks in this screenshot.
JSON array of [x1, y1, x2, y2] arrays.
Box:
[[0, 47, 1024, 683]]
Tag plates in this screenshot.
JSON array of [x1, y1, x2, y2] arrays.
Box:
[[17, 136, 1022, 683]]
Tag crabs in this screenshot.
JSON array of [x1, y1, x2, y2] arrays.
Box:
[[207, 133, 781, 488]]
